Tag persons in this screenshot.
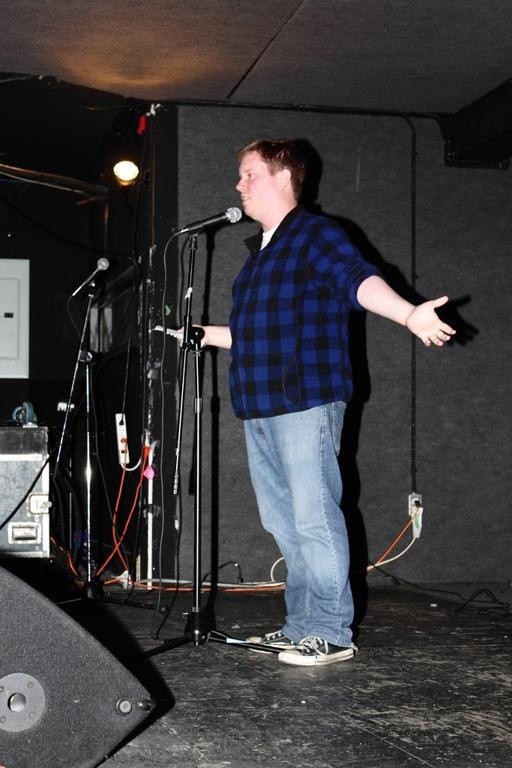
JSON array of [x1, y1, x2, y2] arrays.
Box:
[[179, 137, 458, 668]]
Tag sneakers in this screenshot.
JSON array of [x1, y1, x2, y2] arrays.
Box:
[[277, 634, 354, 667], [246, 629, 298, 654]]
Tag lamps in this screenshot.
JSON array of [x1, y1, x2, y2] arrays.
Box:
[[113, 160, 140, 188]]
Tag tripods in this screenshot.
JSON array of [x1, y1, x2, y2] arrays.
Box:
[[53, 350, 170, 616], [140, 329, 285, 659]]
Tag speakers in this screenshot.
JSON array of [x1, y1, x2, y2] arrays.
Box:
[[443, 113, 511, 169], [0, 566, 167, 768], [0, 426, 51, 560]]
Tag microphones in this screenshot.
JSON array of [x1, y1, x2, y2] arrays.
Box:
[[69, 257, 111, 295], [177, 207, 243, 236]]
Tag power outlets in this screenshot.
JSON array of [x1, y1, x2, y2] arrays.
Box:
[[408, 492, 424, 516]]
